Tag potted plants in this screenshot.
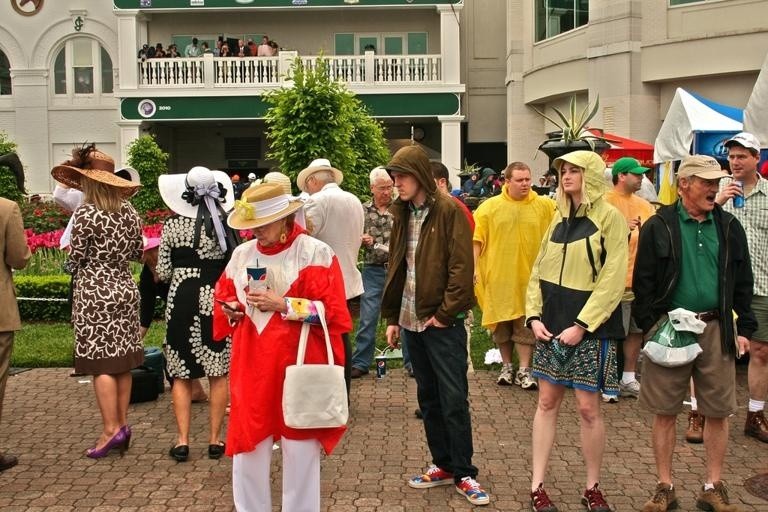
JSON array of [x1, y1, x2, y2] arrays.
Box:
[[452, 158, 482, 184], [527, 92, 624, 174]]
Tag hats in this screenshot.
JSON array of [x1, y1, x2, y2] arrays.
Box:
[[611, 157, 650, 175], [51, 143, 344, 231], [678, 156, 731, 179], [724, 133, 760, 154]]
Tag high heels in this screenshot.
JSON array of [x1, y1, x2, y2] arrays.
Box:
[[86, 425, 131, 457]]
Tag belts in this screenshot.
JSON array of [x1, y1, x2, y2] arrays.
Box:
[[372, 263, 389, 269]]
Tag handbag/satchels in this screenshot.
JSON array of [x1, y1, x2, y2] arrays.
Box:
[[282, 365, 349, 428], [130, 347, 165, 404]]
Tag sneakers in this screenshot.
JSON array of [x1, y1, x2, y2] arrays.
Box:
[[350, 367, 366, 378]]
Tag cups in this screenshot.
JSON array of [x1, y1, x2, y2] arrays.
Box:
[[374, 355, 387, 379], [246, 264, 268, 295]]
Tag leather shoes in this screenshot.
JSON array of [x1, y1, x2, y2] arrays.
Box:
[[0, 454, 17, 470], [208, 441, 225, 459], [169, 444, 188, 460]]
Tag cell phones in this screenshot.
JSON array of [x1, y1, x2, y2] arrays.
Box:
[[216, 298, 237, 310]]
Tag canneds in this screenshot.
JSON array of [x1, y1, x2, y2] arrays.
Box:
[[733, 181, 744, 208]]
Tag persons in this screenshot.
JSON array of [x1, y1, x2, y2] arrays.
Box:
[[153, 146, 490, 512], [632, 133, 768, 511], [466, 150, 658, 512], [51, 146, 144, 458], [0, 196, 32, 470], [133, 35, 279, 85]]
